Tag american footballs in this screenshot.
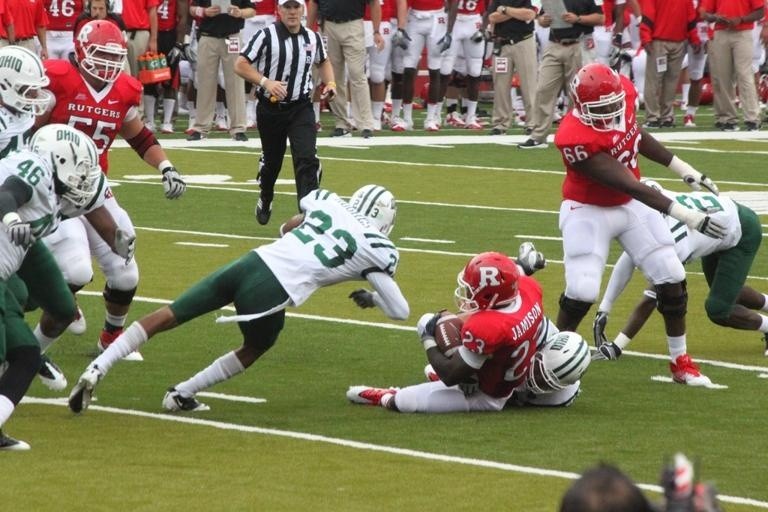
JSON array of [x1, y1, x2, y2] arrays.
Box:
[[435, 309, 464, 359]]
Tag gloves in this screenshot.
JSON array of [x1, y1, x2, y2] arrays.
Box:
[[114, 225, 138, 267], [471, 31, 483, 43], [393, 27, 410, 51], [279, 222, 285, 235], [589, 341, 622, 364], [417, 311, 436, 352], [438, 32, 451, 53], [5, 220, 37, 250], [665, 199, 728, 240], [669, 153, 721, 199], [168, 42, 186, 69], [161, 166, 187, 201], [348, 287, 377, 310], [595, 310, 609, 347]]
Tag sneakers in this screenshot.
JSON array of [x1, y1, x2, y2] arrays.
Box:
[[763, 329, 768, 355], [214, 122, 228, 131], [425, 120, 440, 132], [662, 119, 676, 130], [672, 367, 715, 389], [444, 112, 466, 127], [518, 241, 547, 277], [162, 123, 175, 135], [163, 386, 211, 414], [464, 121, 481, 130], [518, 139, 549, 151], [97, 329, 146, 365], [490, 127, 508, 136], [361, 129, 374, 138], [392, 119, 407, 132], [384, 102, 392, 113], [144, 119, 158, 133], [347, 384, 400, 408], [514, 115, 526, 127], [720, 123, 739, 132], [400, 102, 424, 110], [641, 120, 661, 131], [316, 122, 323, 134], [233, 133, 248, 142], [424, 363, 439, 378], [1, 429, 31, 452], [67, 297, 87, 337], [746, 121, 762, 133], [526, 128, 533, 134], [684, 116, 696, 129], [37, 349, 70, 394], [186, 132, 208, 141], [330, 129, 353, 139], [68, 368, 99, 414], [256, 192, 272, 224]]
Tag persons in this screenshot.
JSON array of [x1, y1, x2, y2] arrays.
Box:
[[558, 453, 722, 511], [304, 0, 378, 137], [518, 1, 605, 151], [68, 186, 411, 417], [73, 1, 130, 49], [598, 3, 625, 79], [0, 123, 100, 452], [594, 177, 768, 360], [38, 18, 184, 361], [242, 0, 275, 127], [232, 0, 338, 226], [685, 43, 709, 129], [401, 0, 445, 133], [122, 1, 161, 134], [699, 0, 767, 132], [187, 19, 228, 130], [489, 0, 542, 134], [42, 1, 77, 60], [622, 6, 654, 112], [4, 0, 50, 59], [347, 1, 406, 129], [157, 0, 187, 135], [639, 1, 694, 128], [553, 65, 712, 385], [348, 240, 544, 411], [189, 3, 253, 143], [456, 239, 591, 405], [38, 125, 134, 393], [1, 45, 48, 367], [435, 1, 487, 129]]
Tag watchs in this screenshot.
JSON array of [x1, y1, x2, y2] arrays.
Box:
[[501, 5, 507, 15], [577, 15, 581, 24]]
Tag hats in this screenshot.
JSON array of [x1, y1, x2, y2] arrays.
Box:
[[280, 0, 308, 9]]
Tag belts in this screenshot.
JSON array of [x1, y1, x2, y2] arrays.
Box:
[[254, 89, 312, 108], [323, 15, 364, 24], [502, 34, 535, 43], [549, 36, 582, 45], [197, 31, 239, 42]]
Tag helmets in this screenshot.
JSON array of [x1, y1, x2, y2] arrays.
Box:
[[75, 20, 129, 83], [455, 252, 519, 312], [29, 124, 103, 221], [0, 44, 57, 116], [348, 184, 395, 236], [570, 63, 626, 131], [700, 84, 714, 104], [639, 175, 664, 197], [525, 329, 591, 394]]
